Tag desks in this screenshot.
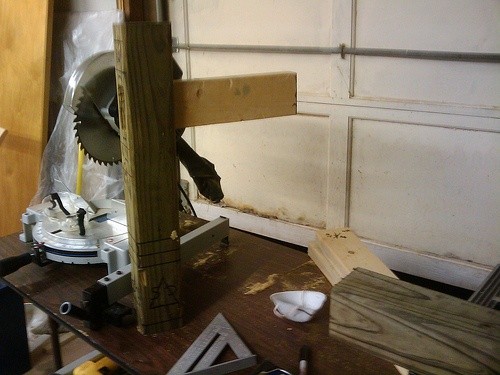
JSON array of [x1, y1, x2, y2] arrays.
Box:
[[0, 214, 500, 375]]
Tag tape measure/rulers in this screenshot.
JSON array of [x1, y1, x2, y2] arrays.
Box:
[[164, 311, 257, 373]]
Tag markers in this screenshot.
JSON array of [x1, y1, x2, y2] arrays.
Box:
[[297, 345, 312, 374]]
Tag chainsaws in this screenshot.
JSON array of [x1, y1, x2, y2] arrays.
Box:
[[70, 60, 225, 328]]
